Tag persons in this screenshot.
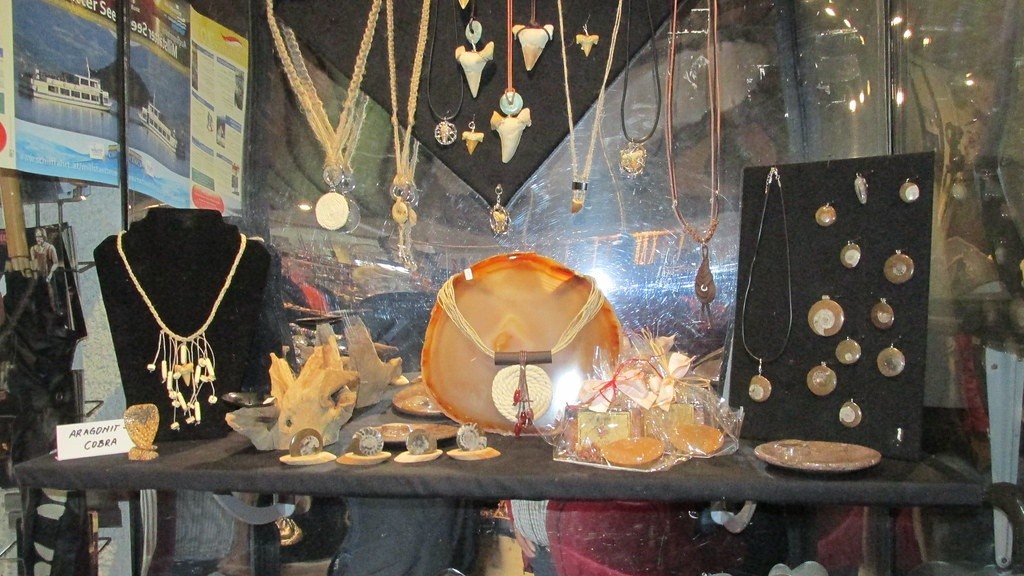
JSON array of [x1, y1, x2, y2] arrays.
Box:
[[30, 228, 58, 313]]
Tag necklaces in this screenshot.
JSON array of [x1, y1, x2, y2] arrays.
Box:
[[437, 272, 604, 434], [265, 0, 721, 305], [117, 228, 246, 430], [742, 166, 792, 403]]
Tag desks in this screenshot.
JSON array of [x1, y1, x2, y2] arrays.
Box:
[[12, 433, 1023, 576]]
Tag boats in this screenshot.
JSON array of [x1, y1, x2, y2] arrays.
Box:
[[19, 56, 113, 112], [138, 90, 178, 152]]
